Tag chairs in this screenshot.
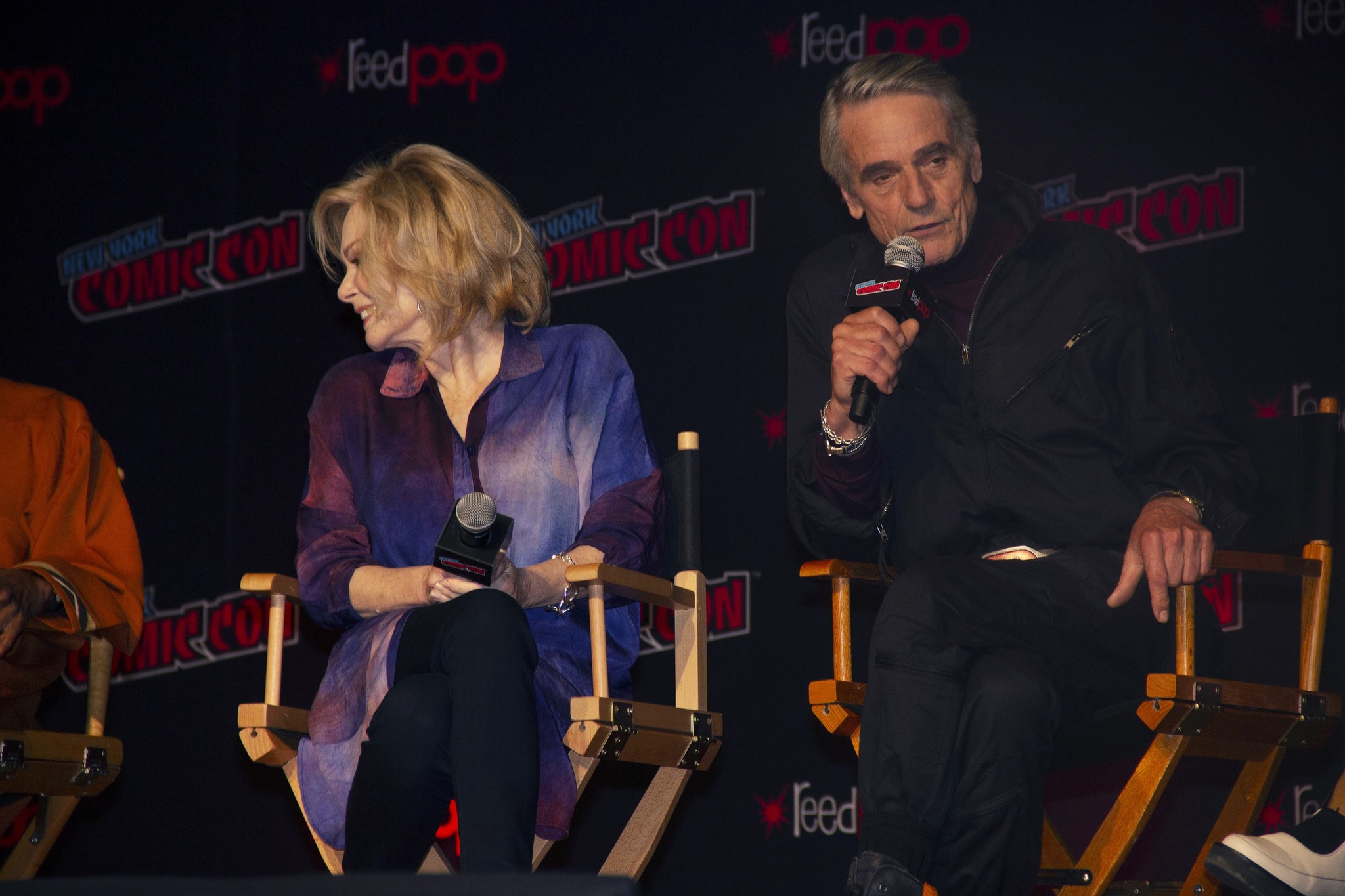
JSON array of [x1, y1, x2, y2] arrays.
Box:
[[234, 431, 719, 886], [0, 464, 129, 882], [800, 532, 1344, 892]]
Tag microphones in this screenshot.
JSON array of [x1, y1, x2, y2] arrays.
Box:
[[431, 491, 516, 589], [842, 235, 927, 426]]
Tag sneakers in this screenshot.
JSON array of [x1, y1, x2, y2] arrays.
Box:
[[1203, 810, 1345, 896]]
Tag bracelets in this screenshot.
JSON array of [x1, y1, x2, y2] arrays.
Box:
[[545, 550, 578, 618], [820, 395, 876, 455]]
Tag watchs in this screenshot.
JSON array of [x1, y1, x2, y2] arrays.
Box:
[[1142, 488, 1207, 527]]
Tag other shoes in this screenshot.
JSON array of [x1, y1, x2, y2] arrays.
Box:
[[841, 850, 928, 896]]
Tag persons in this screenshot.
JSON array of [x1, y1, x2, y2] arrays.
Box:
[[1204, 768, 1345, 896], [782, 49, 1222, 896], [298, 143, 662, 876], [0, 376, 144, 728]]
